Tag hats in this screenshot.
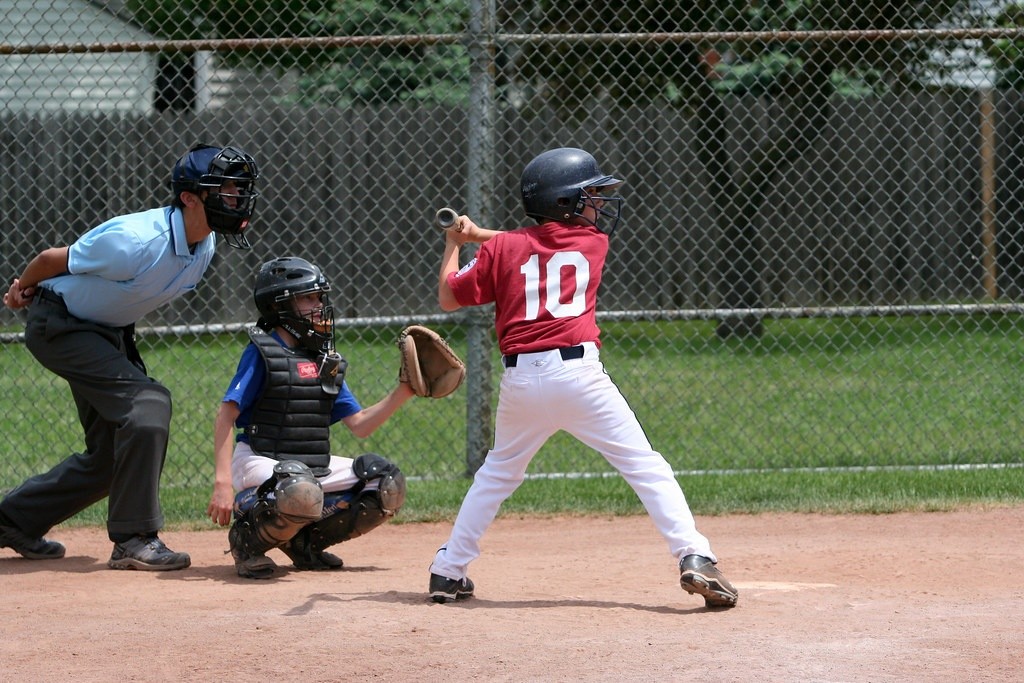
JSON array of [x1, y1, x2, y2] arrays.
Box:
[[172, 146, 249, 183]]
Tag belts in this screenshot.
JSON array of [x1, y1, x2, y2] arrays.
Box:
[[36, 286, 67, 308], [504, 345, 584, 368]]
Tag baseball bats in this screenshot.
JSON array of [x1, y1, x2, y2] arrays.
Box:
[[434, 207, 464, 233]]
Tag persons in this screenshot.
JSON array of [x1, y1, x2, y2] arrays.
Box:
[[207, 258, 467, 579], [0, 143, 258, 571], [429, 147, 738, 608]]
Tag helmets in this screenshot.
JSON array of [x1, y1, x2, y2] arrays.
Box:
[[520, 147, 624, 222], [254, 256, 336, 354]]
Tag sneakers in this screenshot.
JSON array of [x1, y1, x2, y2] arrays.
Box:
[[0, 530, 66, 559], [428, 573, 475, 603], [679, 554, 738, 608], [108, 531, 192, 571], [228, 519, 277, 579], [279, 541, 343, 570]]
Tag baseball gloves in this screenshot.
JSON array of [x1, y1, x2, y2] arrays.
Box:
[[394, 324, 467, 399]]
